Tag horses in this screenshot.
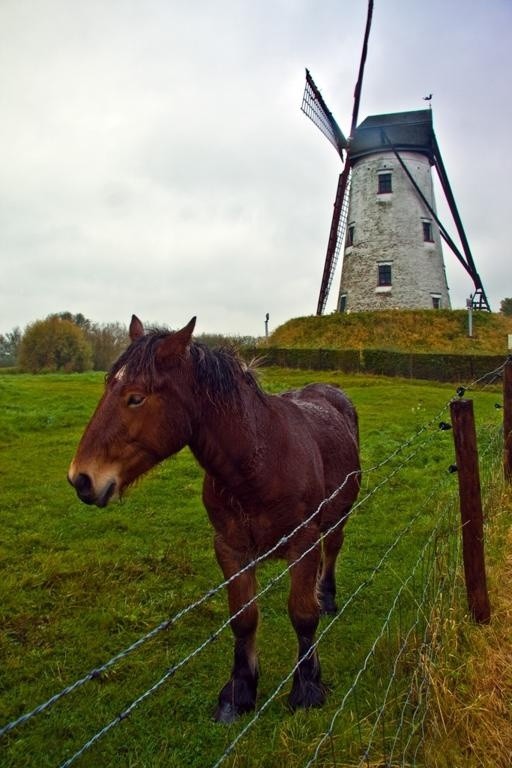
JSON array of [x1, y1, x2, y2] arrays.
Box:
[[66, 312, 363, 723]]
